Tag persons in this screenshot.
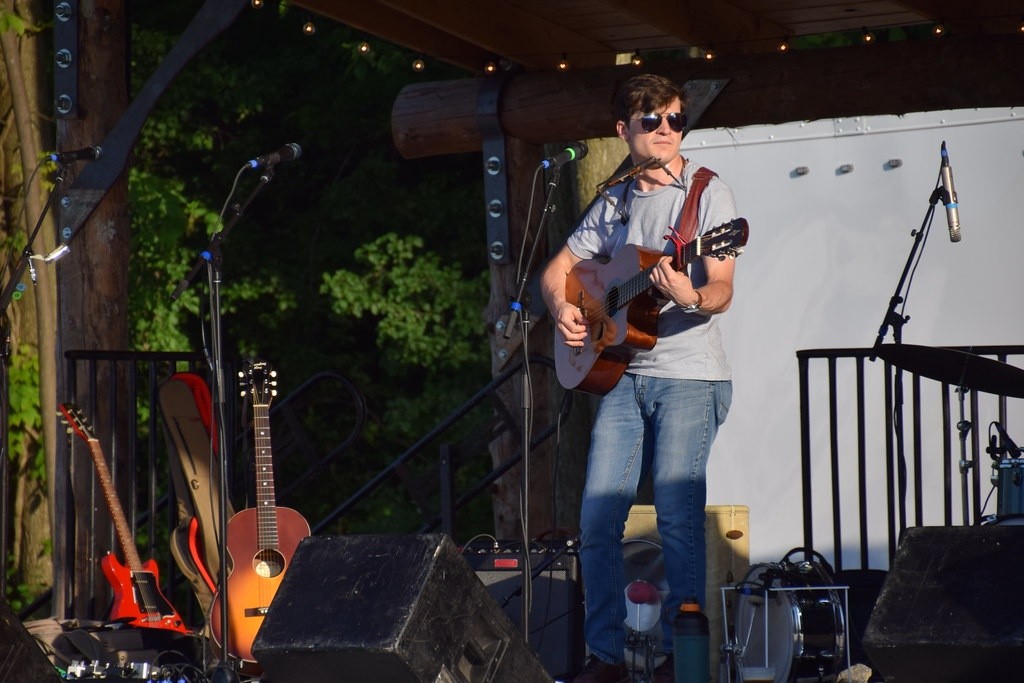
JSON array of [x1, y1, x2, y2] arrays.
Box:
[[539, 76, 737, 681]]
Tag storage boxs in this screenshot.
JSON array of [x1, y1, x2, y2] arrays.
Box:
[[622, 504, 751, 683]]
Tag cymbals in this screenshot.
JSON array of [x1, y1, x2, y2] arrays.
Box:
[[873, 342, 1024, 398]]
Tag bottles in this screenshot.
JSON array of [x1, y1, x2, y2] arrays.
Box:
[[673, 594, 711, 683]]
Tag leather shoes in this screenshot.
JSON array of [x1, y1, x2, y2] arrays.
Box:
[[651, 652, 673, 683], [573, 653, 631, 683]]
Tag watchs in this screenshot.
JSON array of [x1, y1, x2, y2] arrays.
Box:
[[681, 291, 702, 313]]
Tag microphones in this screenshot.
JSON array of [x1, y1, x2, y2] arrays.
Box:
[[994, 420, 1022, 460], [53, 146, 105, 164], [252, 141, 304, 169], [940, 144, 962, 244], [738, 587, 777, 598], [544, 141, 589, 169]]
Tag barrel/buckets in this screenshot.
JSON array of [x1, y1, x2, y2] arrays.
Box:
[[998, 459, 1024, 519]]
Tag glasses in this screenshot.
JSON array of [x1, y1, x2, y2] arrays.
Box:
[[628, 111, 688, 132]]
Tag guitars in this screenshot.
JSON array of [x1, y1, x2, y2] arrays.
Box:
[[206, 356, 313, 679], [54, 399, 190, 640], [553, 218, 750, 398]]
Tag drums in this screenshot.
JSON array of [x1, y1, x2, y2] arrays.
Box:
[[996, 453, 1024, 524], [731, 562, 849, 683]]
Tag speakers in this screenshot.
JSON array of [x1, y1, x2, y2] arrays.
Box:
[[867, 528, 1024, 683], [248, 534, 554, 683]]
